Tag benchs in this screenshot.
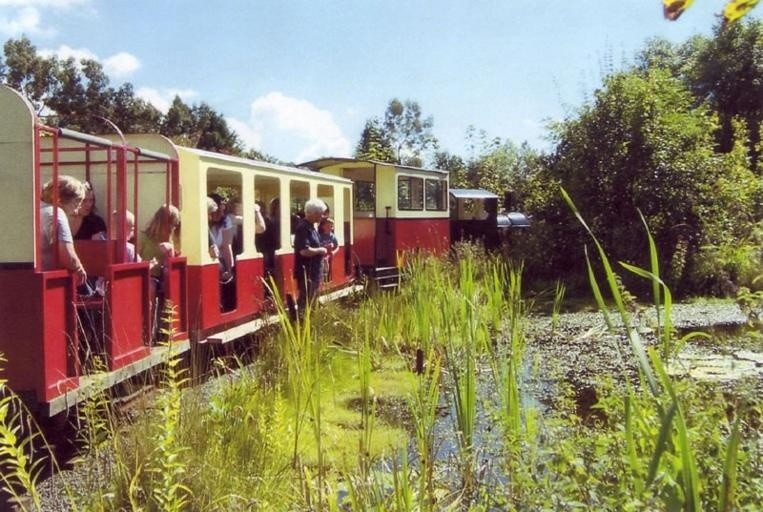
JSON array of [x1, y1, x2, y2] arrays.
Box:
[[70, 239, 128, 351]]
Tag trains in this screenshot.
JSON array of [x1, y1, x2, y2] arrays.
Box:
[[0, 77, 552, 463]]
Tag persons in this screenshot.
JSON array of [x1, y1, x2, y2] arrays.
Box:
[[207, 193, 339, 328], [40, 176, 181, 346]]
